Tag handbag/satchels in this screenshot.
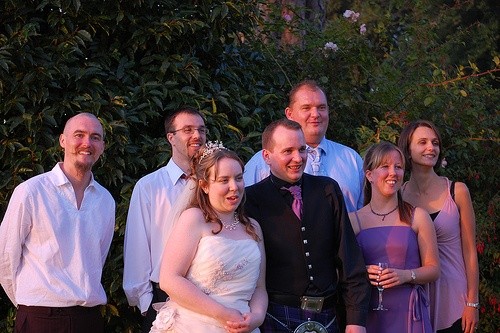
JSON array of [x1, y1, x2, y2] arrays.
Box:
[[293, 318, 327, 333]]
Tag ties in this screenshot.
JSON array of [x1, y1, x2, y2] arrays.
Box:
[[280, 182, 305, 220], [306, 146, 324, 175]]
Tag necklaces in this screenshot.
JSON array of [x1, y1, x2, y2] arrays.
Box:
[[220, 215, 239, 231], [370, 203, 398, 221]]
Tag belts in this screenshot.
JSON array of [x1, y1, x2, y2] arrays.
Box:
[[272, 292, 338, 312]]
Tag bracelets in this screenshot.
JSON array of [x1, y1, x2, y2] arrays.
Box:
[[466, 303, 479, 309], [410, 270, 416, 283]]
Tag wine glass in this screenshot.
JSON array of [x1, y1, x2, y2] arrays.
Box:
[[373, 262, 388, 310]]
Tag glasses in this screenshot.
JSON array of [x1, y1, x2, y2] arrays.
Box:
[[171, 126, 208, 136]]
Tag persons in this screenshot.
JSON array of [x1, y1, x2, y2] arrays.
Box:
[[243, 82, 366, 213], [399, 120, 479, 333], [149, 140, 270, 333], [348, 142, 441, 333], [123, 108, 208, 333], [0, 112, 116, 333], [244, 120, 372, 333]]
